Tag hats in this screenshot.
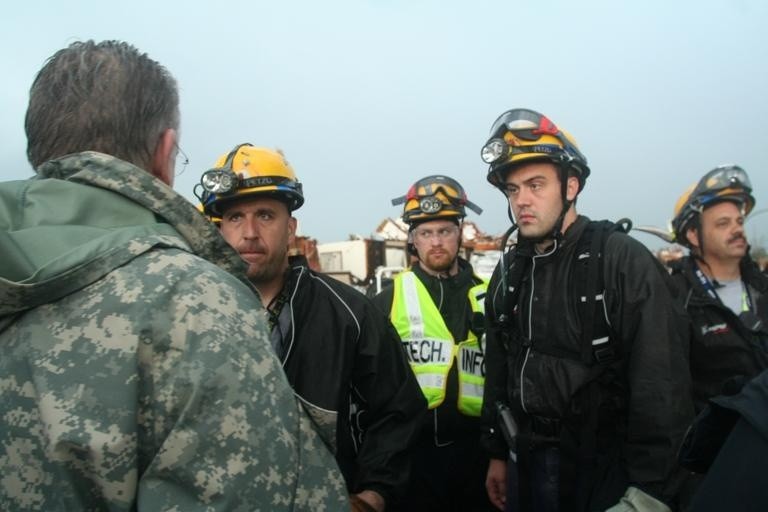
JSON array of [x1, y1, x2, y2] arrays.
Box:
[[201, 143, 305, 219]]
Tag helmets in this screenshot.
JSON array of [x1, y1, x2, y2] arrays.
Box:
[[403, 175, 467, 222], [486, 122, 591, 195], [671, 178, 756, 245]]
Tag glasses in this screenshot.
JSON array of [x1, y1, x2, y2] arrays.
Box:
[[683, 166, 752, 208], [171, 147, 191, 176], [489, 108, 587, 165], [413, 177, 464, 200]]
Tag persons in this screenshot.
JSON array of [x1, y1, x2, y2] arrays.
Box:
[[479, 109, 694, 511], [1, 40, 356, 511], [198, 145, 437, 512], [662, 166, 768, 512], [367, 178, 498, 512]]
